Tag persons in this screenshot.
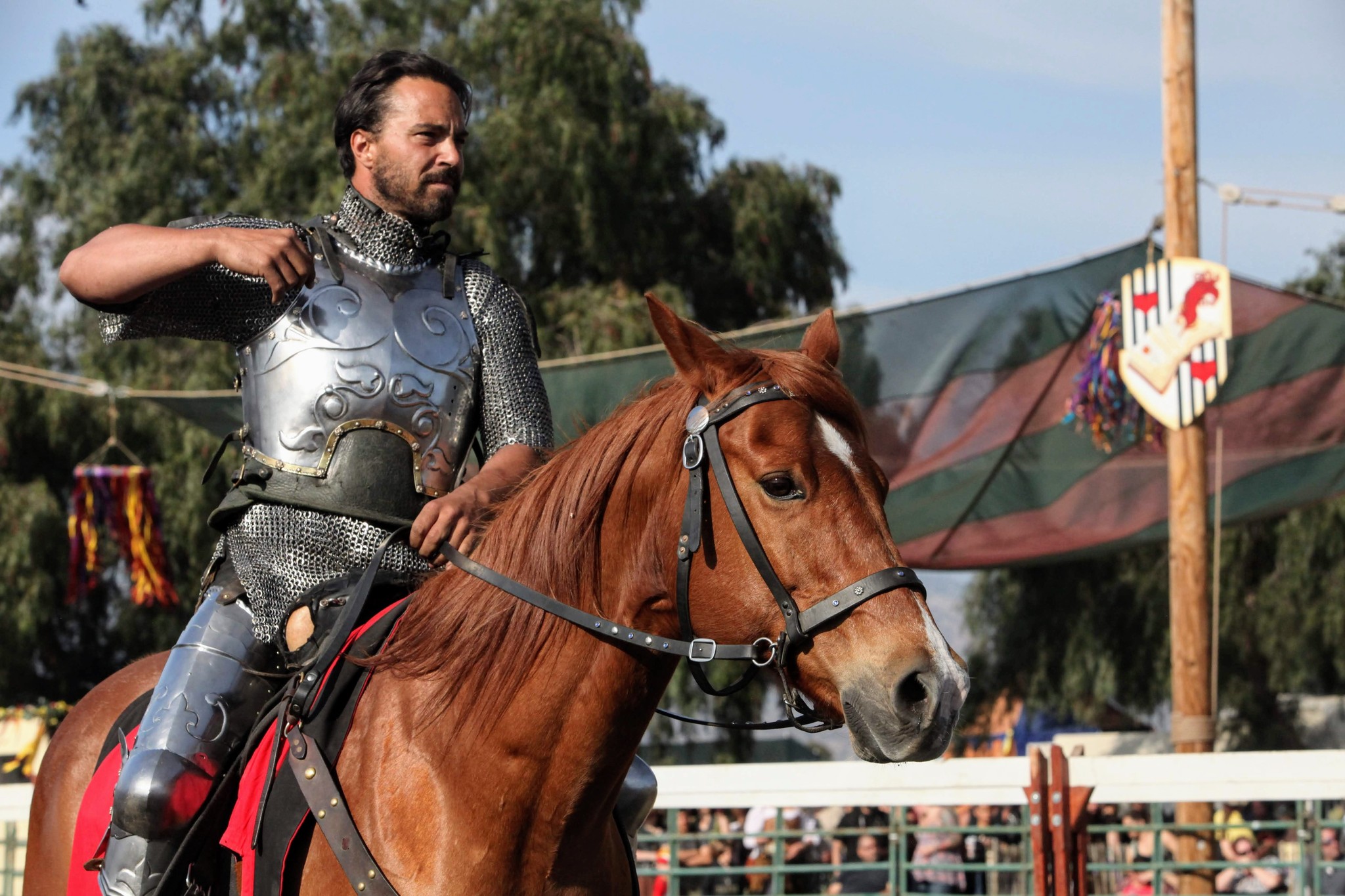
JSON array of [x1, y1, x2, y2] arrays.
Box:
[[622, 804, 1345, 896], [48, 47, 558, 896]]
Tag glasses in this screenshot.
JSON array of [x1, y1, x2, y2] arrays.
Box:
[[1235, 846, 1255, 856], [1322, 834, 1340, 846]]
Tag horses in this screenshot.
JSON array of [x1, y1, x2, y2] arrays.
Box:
[[23, 289, 973, 896]]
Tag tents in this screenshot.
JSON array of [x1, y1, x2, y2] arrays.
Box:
[[118, 231, 1345, 573]]
